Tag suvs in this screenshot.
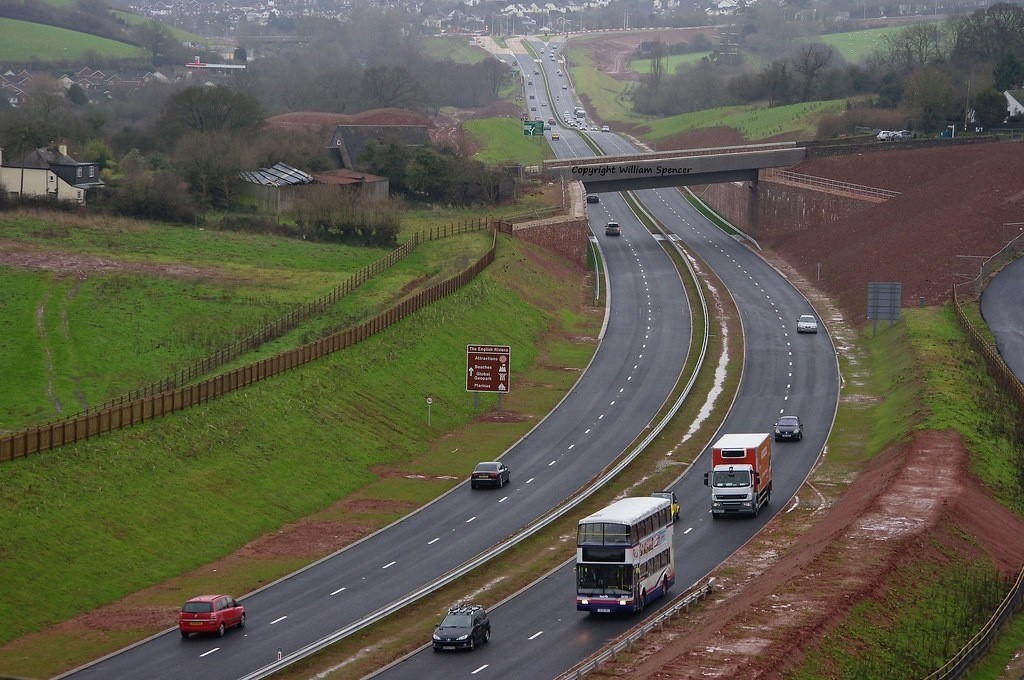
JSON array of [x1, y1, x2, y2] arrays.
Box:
[[431, 602, 493, 651]]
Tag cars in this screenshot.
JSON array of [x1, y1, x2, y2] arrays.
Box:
[[796, 313, 820, 334], [774, 414, 805, 441], [527, 42, 612, 142], [651, 490, 680, 520], [178, 593, 248, 637], [587, 195, 600, 204], [470, 460, 511, 489], [606, 222, 622, 236]]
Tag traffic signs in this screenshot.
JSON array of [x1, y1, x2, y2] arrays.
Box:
[[466, 343, 511, 394]]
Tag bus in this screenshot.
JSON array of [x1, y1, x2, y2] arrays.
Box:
[[575, 494, 676, 619], [703, 431, 776, 519]]
